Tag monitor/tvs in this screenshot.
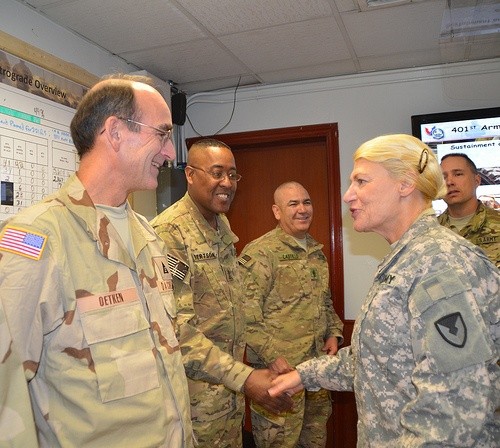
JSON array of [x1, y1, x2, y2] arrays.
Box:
[[410, 107, 500, 220]]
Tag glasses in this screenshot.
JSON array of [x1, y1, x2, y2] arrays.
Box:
[[189, 165, 242, 181], [100, 116, 175, 146]]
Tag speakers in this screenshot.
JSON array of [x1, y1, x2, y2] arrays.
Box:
[[171, 93, 186, 125]]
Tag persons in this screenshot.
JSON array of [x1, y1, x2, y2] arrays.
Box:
[[237, 181, 344, 448], [268, 134, 500, 448], [0, 74, 193, 448], [437, 153, 500, 269], [150, 139, 294, 448]]
